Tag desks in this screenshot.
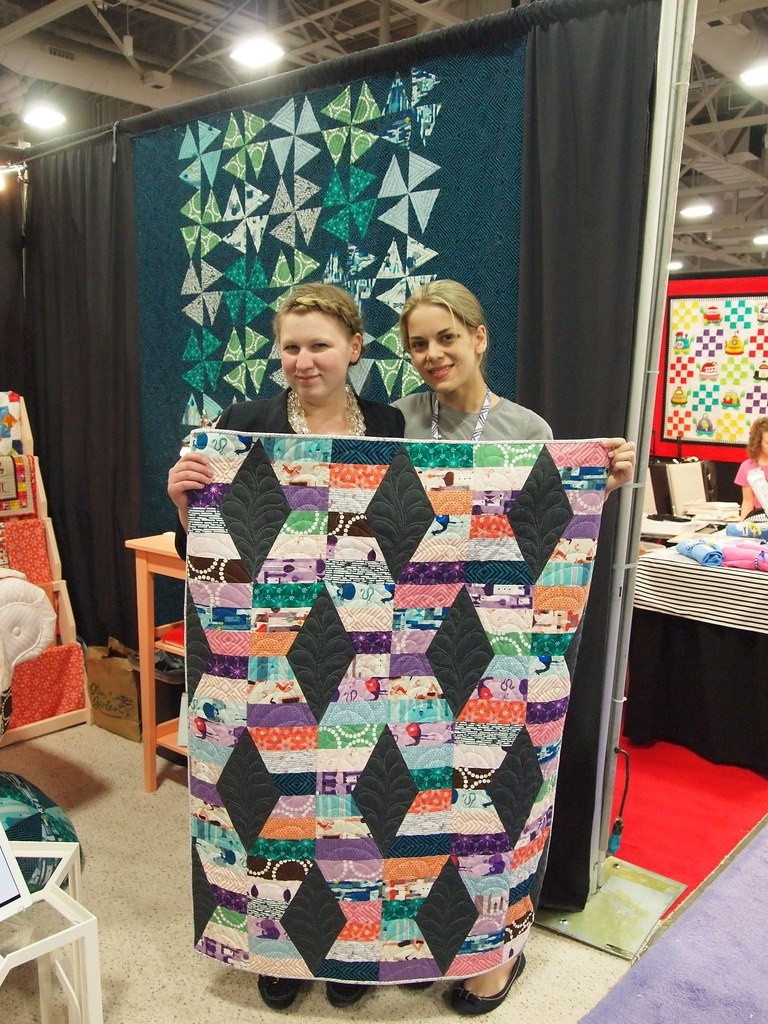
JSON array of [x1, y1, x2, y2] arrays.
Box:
[[0, 841, 101, 1024]]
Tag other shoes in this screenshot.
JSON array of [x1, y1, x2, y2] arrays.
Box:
[[257, 973, 301, 1010], [325, 978, 368, 1007], [450, 952, 526, 1014]]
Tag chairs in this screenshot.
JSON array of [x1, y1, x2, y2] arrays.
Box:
[[640, 469, 717, 541], [667, 461, 741, 526]]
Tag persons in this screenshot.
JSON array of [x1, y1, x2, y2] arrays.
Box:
[[170, 286, 406, 1011], [734, 417, 768, 520], [390, 280, 635, 1015]]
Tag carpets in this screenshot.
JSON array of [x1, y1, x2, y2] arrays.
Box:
[[613, 738, 767, 925]]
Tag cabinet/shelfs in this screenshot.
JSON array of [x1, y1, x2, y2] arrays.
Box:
[[125, 531, 205, 790]]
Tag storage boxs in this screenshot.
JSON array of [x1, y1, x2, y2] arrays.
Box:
[[86, 644, 142, 742]]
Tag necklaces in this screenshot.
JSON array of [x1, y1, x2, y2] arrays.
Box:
[[288, 384, 367, 440], [430, 384, 492, 441]]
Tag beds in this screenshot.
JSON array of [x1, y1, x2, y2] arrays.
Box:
[[623, 514, 768, 778]]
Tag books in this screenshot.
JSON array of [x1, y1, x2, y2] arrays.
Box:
[[666, 462, 742, 524]]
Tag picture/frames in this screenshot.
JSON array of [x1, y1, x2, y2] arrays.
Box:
[[660, 294, 768, 448], [0, 819, 33, 922]]
